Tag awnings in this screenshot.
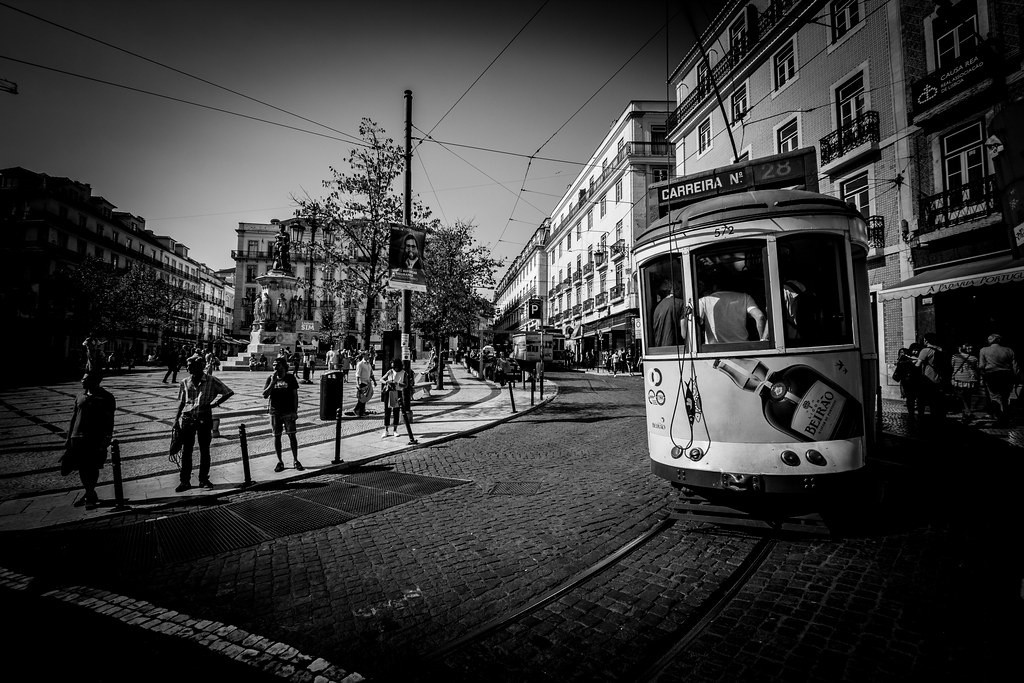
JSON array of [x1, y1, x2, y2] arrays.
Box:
[[878, 248, 1024, 302]]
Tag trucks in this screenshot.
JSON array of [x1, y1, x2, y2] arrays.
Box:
[[509, 331, 553, 361]]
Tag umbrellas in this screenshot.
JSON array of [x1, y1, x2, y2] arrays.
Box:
[[219, 337, 250, 346]]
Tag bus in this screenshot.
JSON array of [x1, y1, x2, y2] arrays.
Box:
[[632, 146, 888, 534]]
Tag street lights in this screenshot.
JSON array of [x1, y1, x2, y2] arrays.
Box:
[[290, 203, 337, 385]]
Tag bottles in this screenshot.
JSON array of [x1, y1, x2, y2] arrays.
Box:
[[712, 357, 863, 443]]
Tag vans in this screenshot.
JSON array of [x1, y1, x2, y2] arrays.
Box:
[[477, 345, 508, 358]]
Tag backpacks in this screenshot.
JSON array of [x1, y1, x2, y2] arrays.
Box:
[[924, 347, 951, 370]]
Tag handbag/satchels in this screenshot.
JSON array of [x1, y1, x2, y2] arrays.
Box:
[[168, 420, 183, 455], [387, 385, 399, 409], [357, 385, 372, 402]]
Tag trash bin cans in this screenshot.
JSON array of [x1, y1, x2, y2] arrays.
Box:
[[319, 370, 344, 421]]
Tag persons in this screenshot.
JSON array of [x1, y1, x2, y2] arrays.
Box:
[[952, 343, 977, 421], [380, 359, 408, 438], [251, 292, 303, 329], [63, 370, 116, 510], [978, 333, 1019, 428], [355, 354, 377, 415], [892, 332, 945, 420], [637, 353, 644, 378], [271, 223, 292, 270], [262, 358, 304, 472], [421, 343, 510, 386], [171, 356, 234, 492], [652, 273, 684, 346], [677, 265, 765, 344], [81, 330, 136, 370], [581, 345, 635, 378], [760, 249, 843, 348], [162, 342, 221, 383], [248, 334, 375, 383]]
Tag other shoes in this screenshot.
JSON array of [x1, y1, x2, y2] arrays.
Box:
[[84, 495, 95, 510], [199, 481, 213, 488], [274, 463, 284, 471], [293, 462, 303, 469], [73, 493, 97, 507], [176, 481, 191, 492], [381, 430, 389, 438], [394, 431, 400, 437]]
